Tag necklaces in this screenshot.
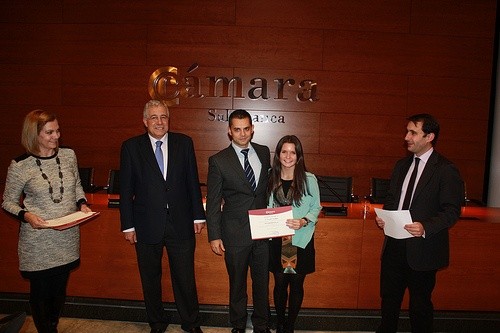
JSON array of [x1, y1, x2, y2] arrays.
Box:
[[36, 153, 64, 203]]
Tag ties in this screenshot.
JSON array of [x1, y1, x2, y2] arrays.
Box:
[[155, 140, 164, 178], [241, 149, 257, 193], [401, 157, 421, 210]]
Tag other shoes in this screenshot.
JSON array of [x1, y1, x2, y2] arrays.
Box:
[[183, 326, 203, 333], [276, 324, 293, 333], [231, 327, 245, 333], [150, 317, 169, 333], [253, 327, 271, 333]]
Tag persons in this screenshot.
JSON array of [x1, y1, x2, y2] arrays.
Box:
[[266, 135, 322, 333], [119, 99, 205, 333], [206, 109, 274, 333], [0, 109, 92, 333], [375, 114, 465, 333]]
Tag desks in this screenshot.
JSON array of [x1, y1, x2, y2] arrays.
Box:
[[0, 192, 500, 333]]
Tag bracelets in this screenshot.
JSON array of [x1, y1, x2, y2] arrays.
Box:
[[302, 218, 308, 227]]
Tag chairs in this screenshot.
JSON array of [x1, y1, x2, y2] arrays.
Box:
[[316, 177, 358, 203], [368, 178, 393, 204], [99, 170, 120, 194], [77, 168, 98, 192]]
